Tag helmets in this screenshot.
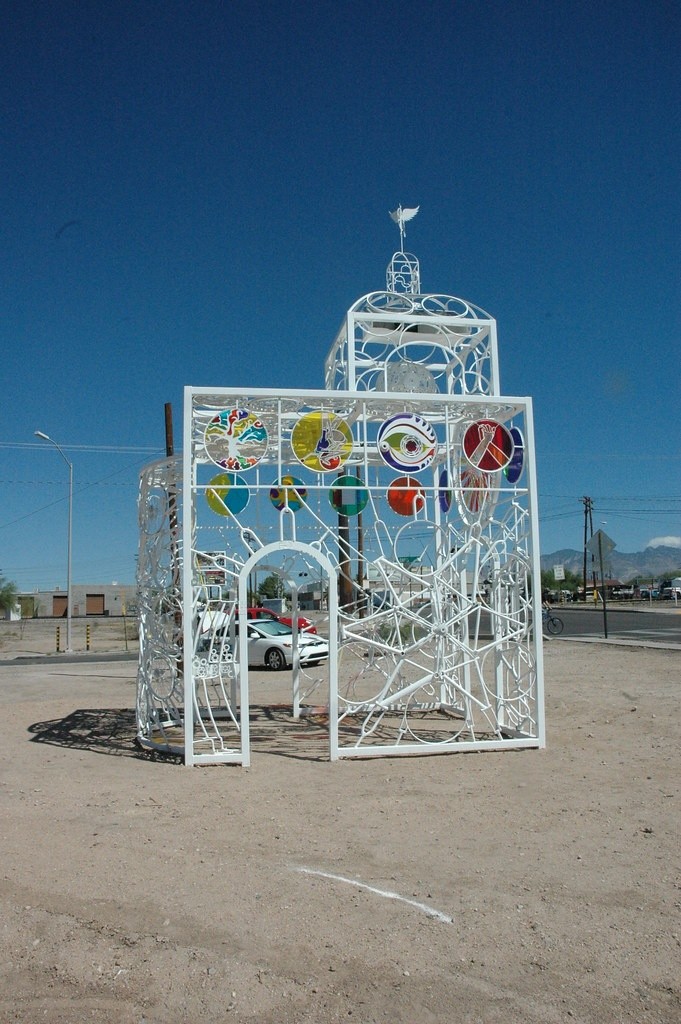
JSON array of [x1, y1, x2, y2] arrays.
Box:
[[544, 588, 550, 592]]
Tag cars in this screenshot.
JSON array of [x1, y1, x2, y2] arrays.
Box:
[[194, 618, 330, 672], [223, 607, 317, 635]]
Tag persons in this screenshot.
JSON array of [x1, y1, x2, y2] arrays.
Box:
[[542, 588, 552, 610]]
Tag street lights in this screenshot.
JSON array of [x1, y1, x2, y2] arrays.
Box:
[[34, 430, 73, 653]]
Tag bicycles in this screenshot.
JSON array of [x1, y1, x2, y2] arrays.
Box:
[[519, 607, 564, 635]]
